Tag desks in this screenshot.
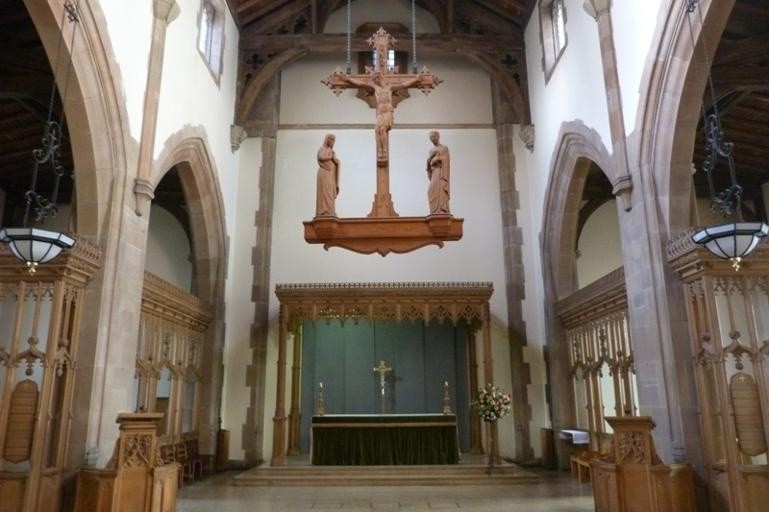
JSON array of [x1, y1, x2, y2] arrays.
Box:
[[310, 411, 463, 467]]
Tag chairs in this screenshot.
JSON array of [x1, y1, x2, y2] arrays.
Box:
[[158, 437, 217, 490]]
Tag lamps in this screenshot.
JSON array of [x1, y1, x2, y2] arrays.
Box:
[[2, 0, 83, 280], [683, 1, 769, 275]]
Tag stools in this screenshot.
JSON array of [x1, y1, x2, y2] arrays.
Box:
[[568, 449, 601, 488]]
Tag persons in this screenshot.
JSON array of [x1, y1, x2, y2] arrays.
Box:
[[426, 130, 450, 213], [342, 72, 422, 156], [315, 132, 340, 216]]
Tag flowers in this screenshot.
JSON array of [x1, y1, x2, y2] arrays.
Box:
[[467, 380, 513, 424]]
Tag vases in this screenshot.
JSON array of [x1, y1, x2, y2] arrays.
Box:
[[483, 419, 502, 465]]
